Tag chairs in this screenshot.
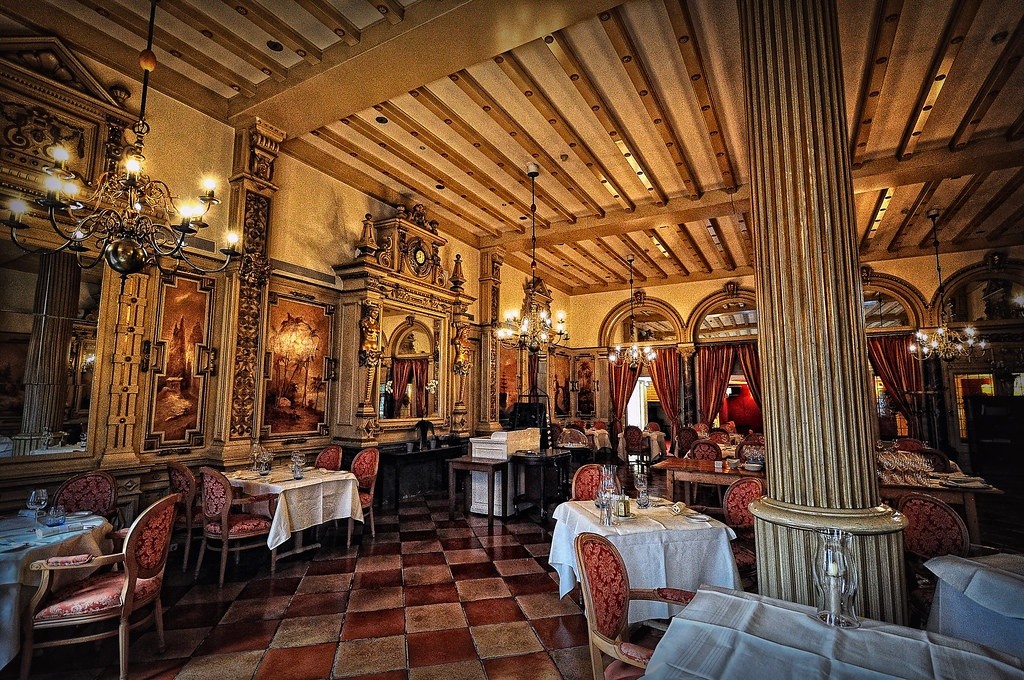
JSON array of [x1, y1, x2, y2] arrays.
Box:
[[0, 401, 969, 680]]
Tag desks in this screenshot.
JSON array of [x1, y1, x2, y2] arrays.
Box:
[[683, 441, 737, 461], [585, 428, 612, 450], [548, 496, 745, 623], [650, 458, 1006, 546], [507, 448, 574, 521], [217, 462, 365, 571], [445, 456, 510, 536], [0, 509, 114, 672], [617, 431, 667, 463], [635, 581, 1024, 680]]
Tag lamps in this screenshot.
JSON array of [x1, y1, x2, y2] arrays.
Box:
[[608, 254, 667, 370], [487, 161, 571, 352], [908, 207, 989, 361], [0, 0, 243, 283]]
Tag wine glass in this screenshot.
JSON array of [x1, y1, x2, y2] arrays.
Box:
[[259, 451, 307, 472], [634, 474, 648, 509], [26, 488, 49, 532]]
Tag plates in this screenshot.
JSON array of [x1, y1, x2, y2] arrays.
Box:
[[302, 466, 314, 471], [65, 511, 92, 518], [685, 513, 712, 522], [612, 512, 636, 520]]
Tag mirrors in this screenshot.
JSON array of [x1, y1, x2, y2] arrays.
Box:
[[380, 304, 447, 423], [0, 238, 104, 464]]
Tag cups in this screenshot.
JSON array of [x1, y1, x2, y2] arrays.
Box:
[[430, 440, 437, 449], [259, 462, 269, 476], [293, 464, 304, 479], [636, 490, 649, 506], [45, 504, 65, 527], [407, 442, 414, 452], [596, 464, 618, 525]]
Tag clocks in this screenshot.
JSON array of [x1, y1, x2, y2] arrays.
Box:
[[415, 249, 426, 264]]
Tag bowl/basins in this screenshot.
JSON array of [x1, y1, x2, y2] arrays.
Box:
[[727, 459, 740, 469], [745, 463, 762, 471]]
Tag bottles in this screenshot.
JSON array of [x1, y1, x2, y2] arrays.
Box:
[[813, 527, 861, 629], [618, 486, 631, 517]]
